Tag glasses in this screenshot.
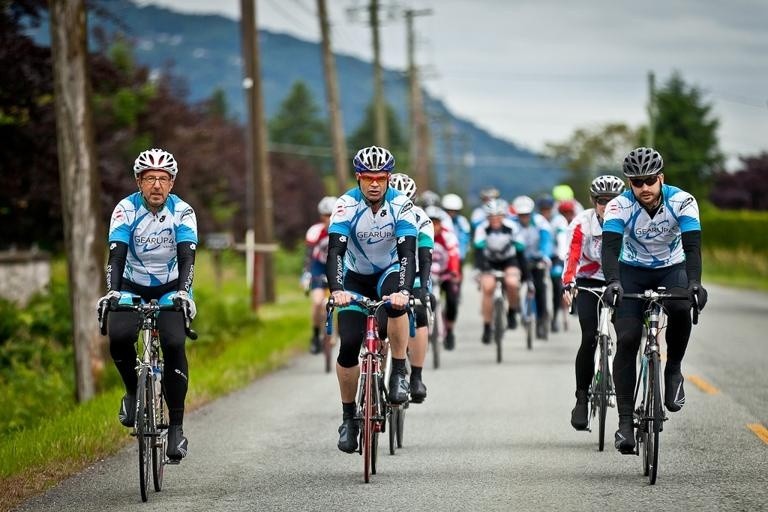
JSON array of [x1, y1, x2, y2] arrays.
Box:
[[358, 174, 388, 186], [596, 199, 609, 205], [630, 176, 658, 188], [143, 176, 168, 185]]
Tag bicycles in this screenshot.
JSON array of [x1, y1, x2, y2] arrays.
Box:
[[99, 296, 198, 502], [327, 296, 415, 483], [364, 295, 434, 455], [612, 285, 699, 485], [304, 262, 581, 372], [568, 282, 631, 453]]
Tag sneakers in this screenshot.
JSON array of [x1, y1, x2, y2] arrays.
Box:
[[442, 331, 455, 350], [338, 415, 360, 454], [507, 312, 517, 329], [409, 373, 426, 399], [118, 394, 136, 427], [388, 371, 410, 404], [308, 334, 320, 354], [166, 425, 188, 460], [570, 389, 590, 430], [614, 415, 635, 450], [664, 362, 685, 412], [481, 327, 492, 344]]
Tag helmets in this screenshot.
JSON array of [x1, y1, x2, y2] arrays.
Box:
[[133, 148, 178, 182], [388, 173, 418, 203], [589, 174, 626, 198], [317, 196, 337, 215], [416, 185, 574, 222], [353, 145, 395, 173], [622, 146, 664, 177]]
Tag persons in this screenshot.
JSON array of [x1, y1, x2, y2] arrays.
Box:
[[563, 148, 707, 456], [565, 175, 626, 425], [97, 148, 199, 459], [301, 145, 470, 354], [326, 145, 418, 455], [472, 183, 586, 344], [388, 174, 435, 402]]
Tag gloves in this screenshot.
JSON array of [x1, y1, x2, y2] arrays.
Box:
[[686, 281, 708, 309], [602, 281, 623, 308], [95, 291, 122, 321], [412, 287, 436, 312], [173, 289, 198, 321]]
